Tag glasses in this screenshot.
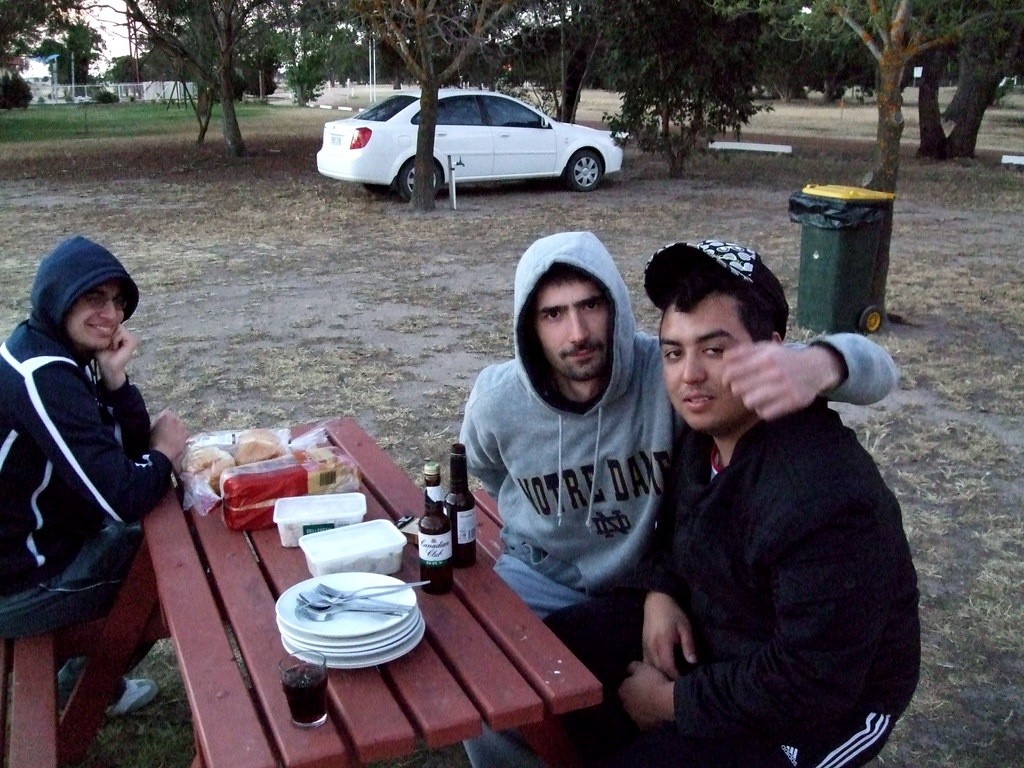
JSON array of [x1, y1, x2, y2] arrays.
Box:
[[83, 289, 128, 311]]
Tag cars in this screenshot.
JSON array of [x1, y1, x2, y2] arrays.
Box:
[[317, 88, 623, 200]]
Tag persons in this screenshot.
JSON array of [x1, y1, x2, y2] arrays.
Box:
[[1, 236, 190, 716], [459, 230, 897, 768], [618, 239, 921, 768]]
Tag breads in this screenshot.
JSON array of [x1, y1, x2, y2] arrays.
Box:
[[183, 427, 336, 533]]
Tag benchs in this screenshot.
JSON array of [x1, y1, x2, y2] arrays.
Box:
[[0, 630, 60, 767], [473, 488, 504, 568]]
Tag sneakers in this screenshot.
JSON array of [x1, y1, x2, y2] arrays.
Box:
[[57, 659, 158, 717]]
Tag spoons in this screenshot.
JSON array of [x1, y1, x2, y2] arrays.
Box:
[[293, 591, 415, 613]]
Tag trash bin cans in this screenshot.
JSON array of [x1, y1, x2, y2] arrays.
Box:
[[789, 182, 897, 335]]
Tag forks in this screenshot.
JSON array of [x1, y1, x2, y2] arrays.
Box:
[[314, 580, 431, 597], [296, 606, 412, 622]]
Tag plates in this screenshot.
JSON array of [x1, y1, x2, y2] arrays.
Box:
[[275, 571, 426, 669]]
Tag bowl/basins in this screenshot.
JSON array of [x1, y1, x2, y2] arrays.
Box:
[[273, 492, 367, 547], [298, 518, 407, 577]]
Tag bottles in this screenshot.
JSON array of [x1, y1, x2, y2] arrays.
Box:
[[417, 462, 454, 595], [444, 444, 477, 568]]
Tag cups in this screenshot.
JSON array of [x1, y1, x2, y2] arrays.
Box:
[[278, 650, 328, 728]]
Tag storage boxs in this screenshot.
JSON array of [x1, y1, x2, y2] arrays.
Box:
[[299, 519, 407, 578], [272, 491, 368, 548]]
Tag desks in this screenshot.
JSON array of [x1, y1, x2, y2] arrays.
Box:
[[60, 419, 602, 768]]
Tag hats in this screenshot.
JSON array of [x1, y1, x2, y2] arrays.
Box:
[[643, 237, 789, 341]]
